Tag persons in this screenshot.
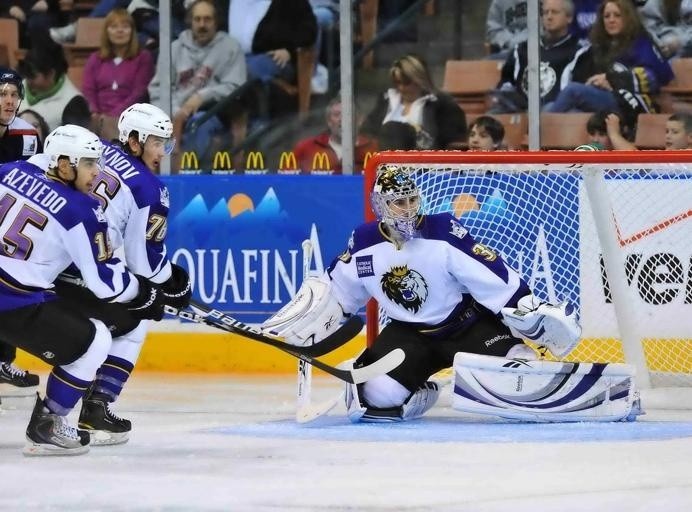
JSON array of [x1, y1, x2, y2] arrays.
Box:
[[0, 70, 42, 388], [260, 172, 638, 424], [55, 103, 192, 432], [1, 125, 165, 450]]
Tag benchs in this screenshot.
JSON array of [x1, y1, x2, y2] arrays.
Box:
[[423, 60, 691, 154]]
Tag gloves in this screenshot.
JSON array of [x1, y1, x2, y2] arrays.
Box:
[[125, 262, 190, 320]]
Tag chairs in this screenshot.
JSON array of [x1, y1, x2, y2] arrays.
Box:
[[0, 0, 379, 168]]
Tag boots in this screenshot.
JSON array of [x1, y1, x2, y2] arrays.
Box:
[[0, 365, 131, 448]]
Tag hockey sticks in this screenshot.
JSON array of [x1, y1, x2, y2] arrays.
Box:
[[190, 302, 405, 384], [295, 359, 344, 424], [58, 273, 363, 357]]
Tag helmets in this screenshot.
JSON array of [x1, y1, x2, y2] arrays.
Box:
[[44, 124, 101, 169], [0, 68, 24, 100], [371, 171, 422, 240], [118, 103, 173, 144]]
